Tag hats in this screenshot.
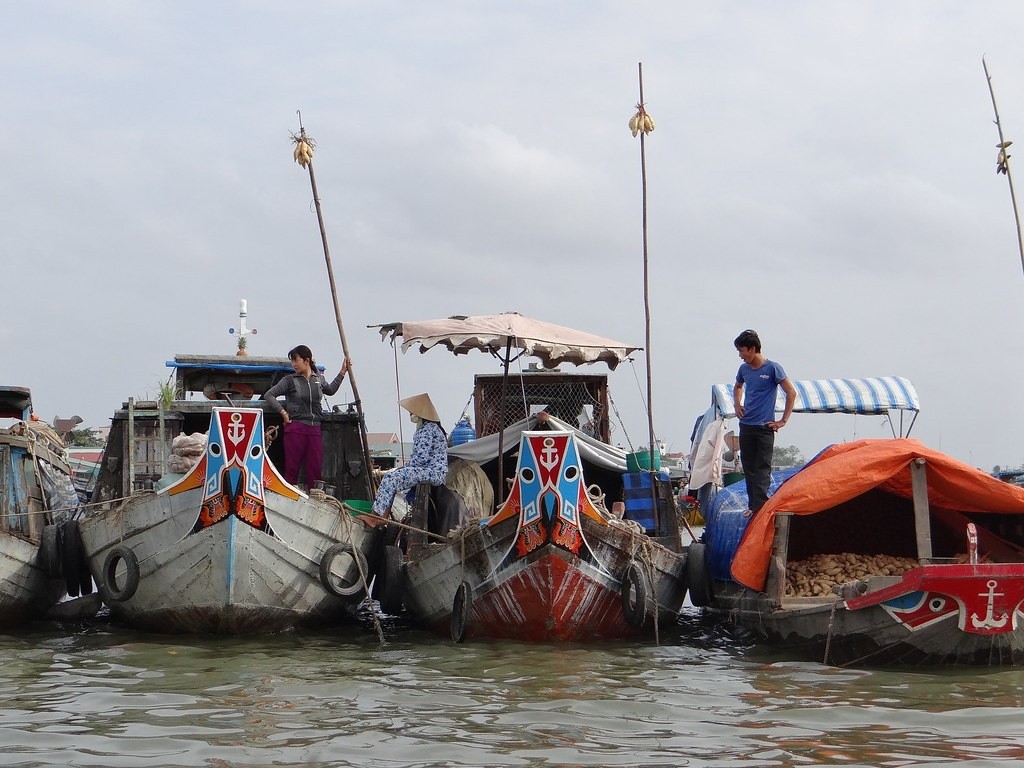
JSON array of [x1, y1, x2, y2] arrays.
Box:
[[397, 393, 440, 422]]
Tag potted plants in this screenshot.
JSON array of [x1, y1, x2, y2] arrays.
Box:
[[155, 379, 183, 411], [237, 337, 247, 357]]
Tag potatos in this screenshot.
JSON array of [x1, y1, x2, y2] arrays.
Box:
[[784, 550, 920, 596], [167, 432, 208, 474]]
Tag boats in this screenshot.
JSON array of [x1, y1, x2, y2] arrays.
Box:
[[366, 311, 691, 648], [687, 377, 1024, 671], [0, 382, 83, 626], [74, 298, 396, 639]]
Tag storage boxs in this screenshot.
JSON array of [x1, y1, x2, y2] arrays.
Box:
[[343, 500, 373, 517], [623, 472, 671, 534], [155, 474, 185, 492]]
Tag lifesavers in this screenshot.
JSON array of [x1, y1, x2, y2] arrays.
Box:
[[38, 520, 93, 597], [826, 631, 886, 669], [687, 541, 714, 607], [319, 541, 369, 599], [450, 581, 473, 644], [620, 564, 646, 628], [102, 544, 141, 603]]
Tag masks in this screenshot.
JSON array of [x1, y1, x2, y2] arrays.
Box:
[[410, 413, 419, 423]]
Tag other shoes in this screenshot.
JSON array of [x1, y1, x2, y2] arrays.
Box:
[[742, 510, 752, 518]]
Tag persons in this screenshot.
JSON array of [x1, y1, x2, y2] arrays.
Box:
[[733, 329, 797, 516], [355, 393, 448, 528], [674, 476, 699, 515], [264, 345, 352, 493]]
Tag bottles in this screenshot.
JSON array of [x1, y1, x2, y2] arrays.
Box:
[[452, 420, 475, 446]]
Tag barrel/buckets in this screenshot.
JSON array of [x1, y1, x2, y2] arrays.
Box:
[[626, 450, 660, 472]]
